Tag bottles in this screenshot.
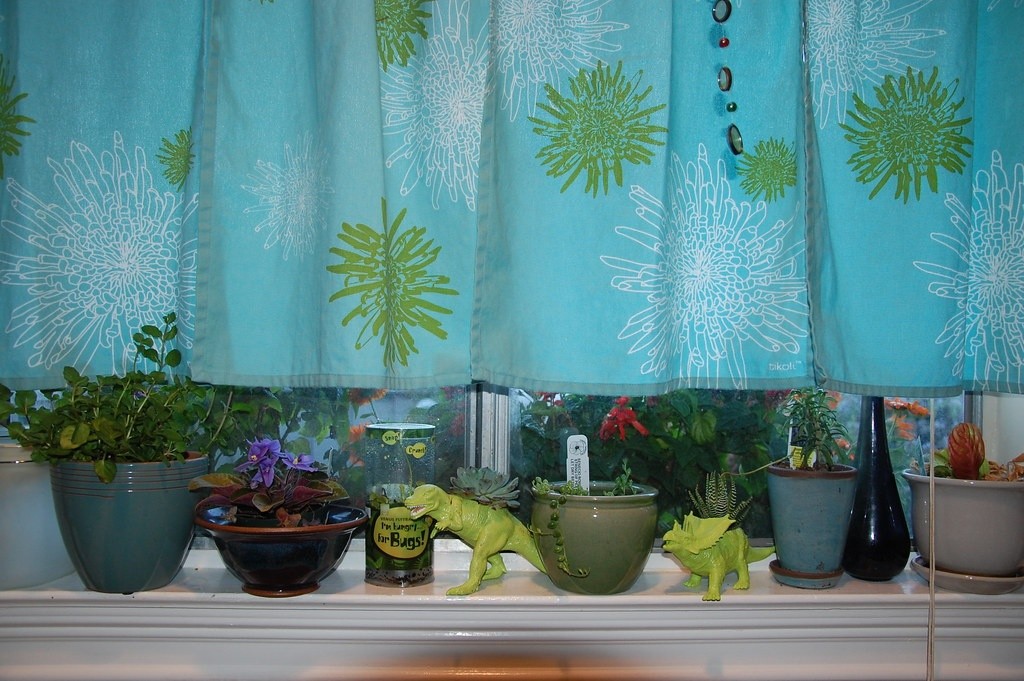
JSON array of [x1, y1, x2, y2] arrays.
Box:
[[842, 396, 911, 581]]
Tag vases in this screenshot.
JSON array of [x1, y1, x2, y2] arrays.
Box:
[[193, 504, 369, 598]]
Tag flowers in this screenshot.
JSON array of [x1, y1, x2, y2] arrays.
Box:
[[189, 437, 349, 527]]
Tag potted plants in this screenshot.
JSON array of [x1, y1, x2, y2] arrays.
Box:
[[901, 422, 1024, 594], [404, 467, 548, 595], [662, 468, 777, 601], [766, 385, 858, 590], [532, 472, 660, 596], [0, 313, 281, 594]]
